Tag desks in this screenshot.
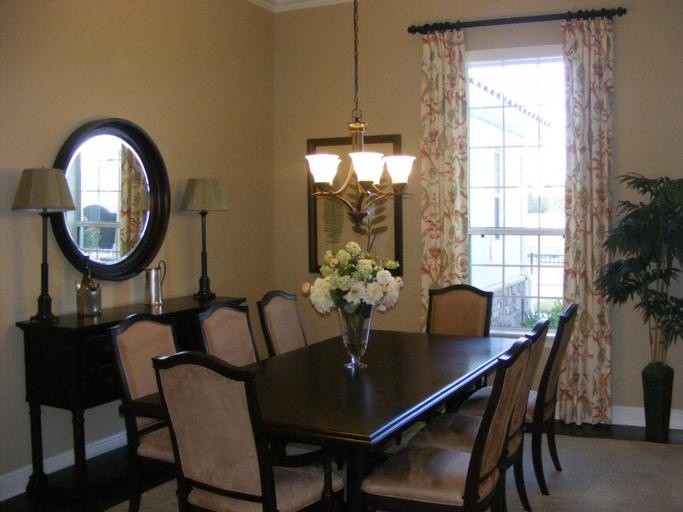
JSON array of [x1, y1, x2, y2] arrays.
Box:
[[118, 329, 519, 510], [16, 295, 247, 504]]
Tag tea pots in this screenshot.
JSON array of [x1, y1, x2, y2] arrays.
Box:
[[73, 256, 102, 316], [144, 260, 166, 306]]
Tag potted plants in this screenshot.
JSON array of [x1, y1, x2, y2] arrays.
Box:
[[592, 172, 683, 444]]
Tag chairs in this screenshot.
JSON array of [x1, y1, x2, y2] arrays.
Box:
[[150, 349, 344, 510], [196, 302, 259, 368], [359, 317, 550, 510], [256, 289, 309, 357], [107, 313, 193, 512], [458, 302, 578, 495], [425, 285, 495, 338]]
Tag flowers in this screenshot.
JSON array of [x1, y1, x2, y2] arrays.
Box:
[[301, 240, 404, 316]]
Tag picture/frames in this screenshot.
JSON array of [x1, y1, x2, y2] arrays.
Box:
[[307, 134, 403, 278]]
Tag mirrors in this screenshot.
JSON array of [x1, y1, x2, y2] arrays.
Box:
[[50, 118, 171, 281]]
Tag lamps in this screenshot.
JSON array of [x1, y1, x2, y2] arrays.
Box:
[[178, 176, 231, 302], [11, 165, 77, 323], [304, 0, 416, 224]]
